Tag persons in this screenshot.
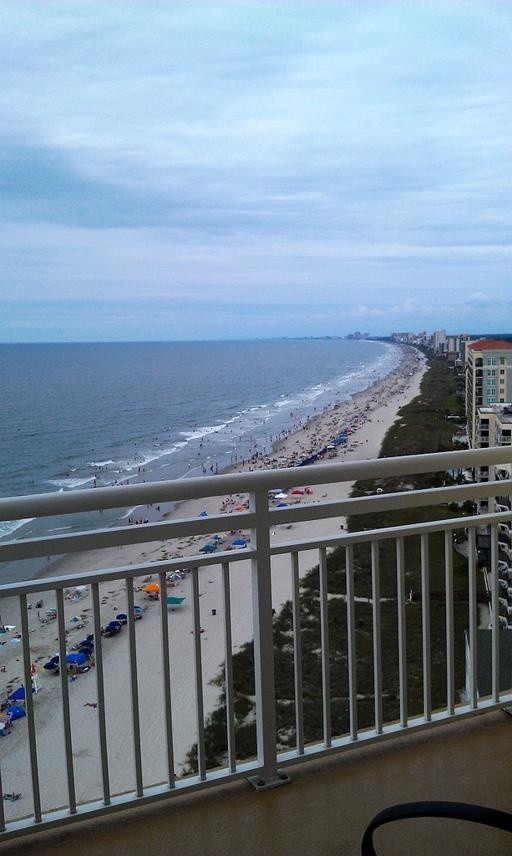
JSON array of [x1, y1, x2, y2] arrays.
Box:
[[0, 360, 409, 734]]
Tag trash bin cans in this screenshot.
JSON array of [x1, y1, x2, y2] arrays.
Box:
[[341, 525, 343, 529], [212, 609, 216, 615]]
